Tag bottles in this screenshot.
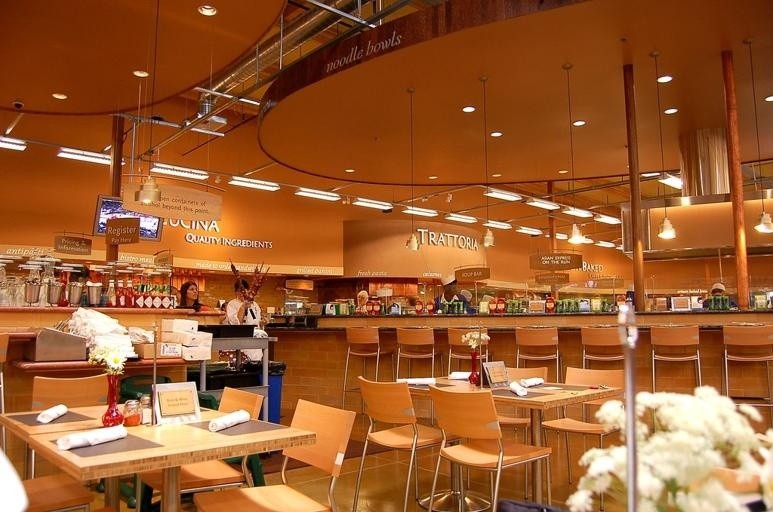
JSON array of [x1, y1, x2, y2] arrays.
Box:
[[710, 295, 730, 310], [106, 279, 134, 307], [365, 302, 385, 316], [135, 280, 169, 293], [490, 298, 523, 315], [546, 295, 579, 313], [442, 301, 464, 314], [122, 394, 153, 427], [415, 299, 435, 315]]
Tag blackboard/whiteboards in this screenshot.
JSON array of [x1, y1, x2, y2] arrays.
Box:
[[455, 268, 490, 282], [529, 253, 582, 270], [105, 218, 140, 244], [122, 182, 223, 221], [54, 236, 92, 254], [535, 273, 569, 285]]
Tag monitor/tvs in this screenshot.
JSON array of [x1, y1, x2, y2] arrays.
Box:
[[93, 194, 164, 243]]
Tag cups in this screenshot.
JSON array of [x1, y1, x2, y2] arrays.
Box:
[[22, 280, 103, 305]]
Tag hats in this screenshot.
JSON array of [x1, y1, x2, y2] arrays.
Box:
[[440, 274, 456, 287], [710, 283, 726, 292]]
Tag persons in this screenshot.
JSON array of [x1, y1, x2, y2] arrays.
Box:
[[700, 281, 739, 307], [224, 278, 269, 331], [175, 280, 201, 311], [431, 274, 471, 314]]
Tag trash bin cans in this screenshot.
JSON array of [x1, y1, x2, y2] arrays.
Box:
[[243, 359, 286, 454]]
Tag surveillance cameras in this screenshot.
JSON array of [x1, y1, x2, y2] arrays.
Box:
[[12, 101, 25, 110]]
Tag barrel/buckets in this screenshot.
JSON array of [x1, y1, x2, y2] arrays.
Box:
[[591, 298, 603, 312], [577, 299, 590, 312]]
